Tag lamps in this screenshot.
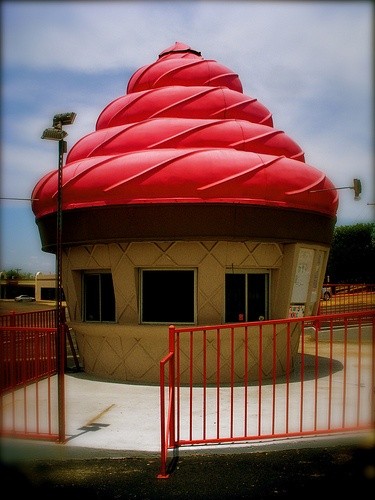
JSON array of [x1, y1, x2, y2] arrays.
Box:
[[319, 178, 364, 202]]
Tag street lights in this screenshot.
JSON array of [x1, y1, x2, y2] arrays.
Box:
[[40, 113, 86, 374]]
